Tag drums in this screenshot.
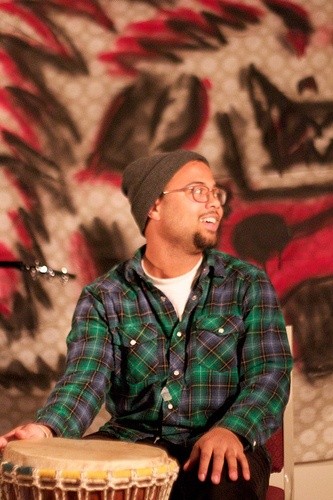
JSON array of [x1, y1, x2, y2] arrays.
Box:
[[0, 436, 181, 500]]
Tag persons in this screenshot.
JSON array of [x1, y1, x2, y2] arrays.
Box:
[[1, 151, 297, 499]]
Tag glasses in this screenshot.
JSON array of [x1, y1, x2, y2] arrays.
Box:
[[161, 185, 226, 205]]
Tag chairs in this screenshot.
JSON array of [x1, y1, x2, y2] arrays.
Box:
[[262, 325, 294, 500]]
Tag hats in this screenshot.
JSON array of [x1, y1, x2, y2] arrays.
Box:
[[121, 151, 210, 236]]
